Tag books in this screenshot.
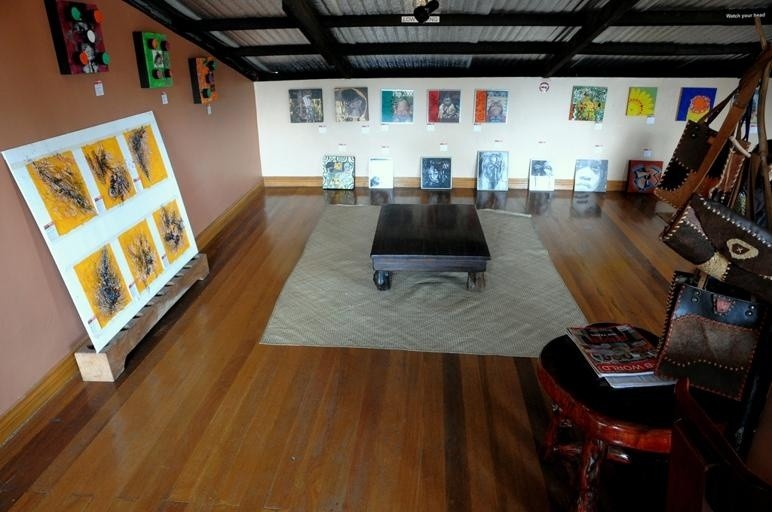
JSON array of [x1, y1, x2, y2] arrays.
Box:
[[605, 375, 678, 389], [563, 323, 658, 378]]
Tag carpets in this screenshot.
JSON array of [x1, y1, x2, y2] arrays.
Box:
[[254, 200, 589, 361]]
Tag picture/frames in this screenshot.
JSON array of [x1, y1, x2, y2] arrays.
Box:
[[419, 156, 453, 190], [379, 88, 509, 126], [476, 150, 509, 193]]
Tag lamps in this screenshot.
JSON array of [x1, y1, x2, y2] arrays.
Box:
[[411, 0, 441, 26]]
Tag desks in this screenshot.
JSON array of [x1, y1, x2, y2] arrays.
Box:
[[533, 317, 673, 509], [366, 202, 495, 294]]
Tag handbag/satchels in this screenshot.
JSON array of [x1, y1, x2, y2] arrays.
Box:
[[654, 119, 772, 402]]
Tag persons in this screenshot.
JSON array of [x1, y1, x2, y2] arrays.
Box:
[[289, 89, 457, 203], [574, 160, 604, 192]]
